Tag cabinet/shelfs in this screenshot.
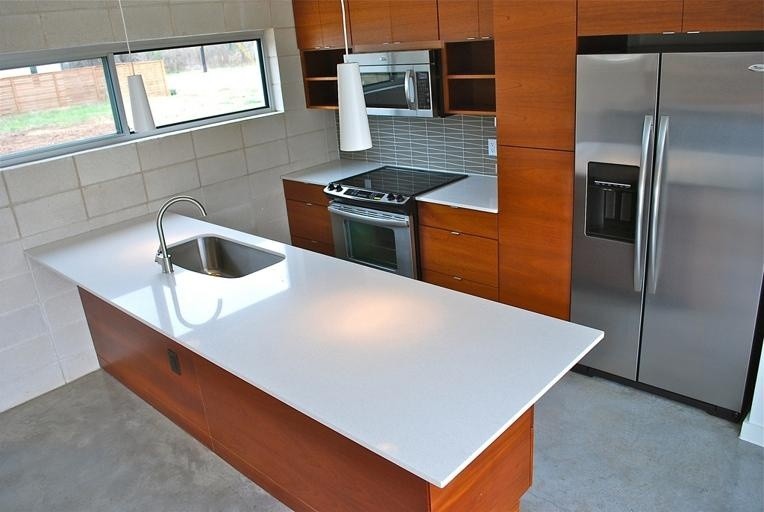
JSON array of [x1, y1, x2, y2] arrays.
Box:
[[436, 0, 492, 44], [412, 201, 498, 302], [298, 44, 352, 112], [441, 36, 496, 120], [345, 0, 439, 54], [492, 1, 577, 331], [577, 1, 762, 39], [289, 1, 346, 51], [282, 178, 331, 253]]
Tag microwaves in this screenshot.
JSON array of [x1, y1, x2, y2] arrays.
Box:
[[341, 51, 443, 119]]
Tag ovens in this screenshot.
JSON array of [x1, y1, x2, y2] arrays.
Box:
[[327, 203, 416, 280]]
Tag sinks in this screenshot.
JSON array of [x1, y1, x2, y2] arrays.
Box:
[[160, 232, 287, 279]]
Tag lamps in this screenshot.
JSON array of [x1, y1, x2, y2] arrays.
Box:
[[332, 0, 375, 157], [114, 1, 156, 140]]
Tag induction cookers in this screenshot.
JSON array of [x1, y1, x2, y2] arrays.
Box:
[[323, 165, 470, 215]]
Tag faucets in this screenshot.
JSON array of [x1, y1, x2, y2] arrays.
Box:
[[155, 195, 207, 274]]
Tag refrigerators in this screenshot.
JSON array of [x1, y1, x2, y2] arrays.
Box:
[[568, 31, 762, 425]]
